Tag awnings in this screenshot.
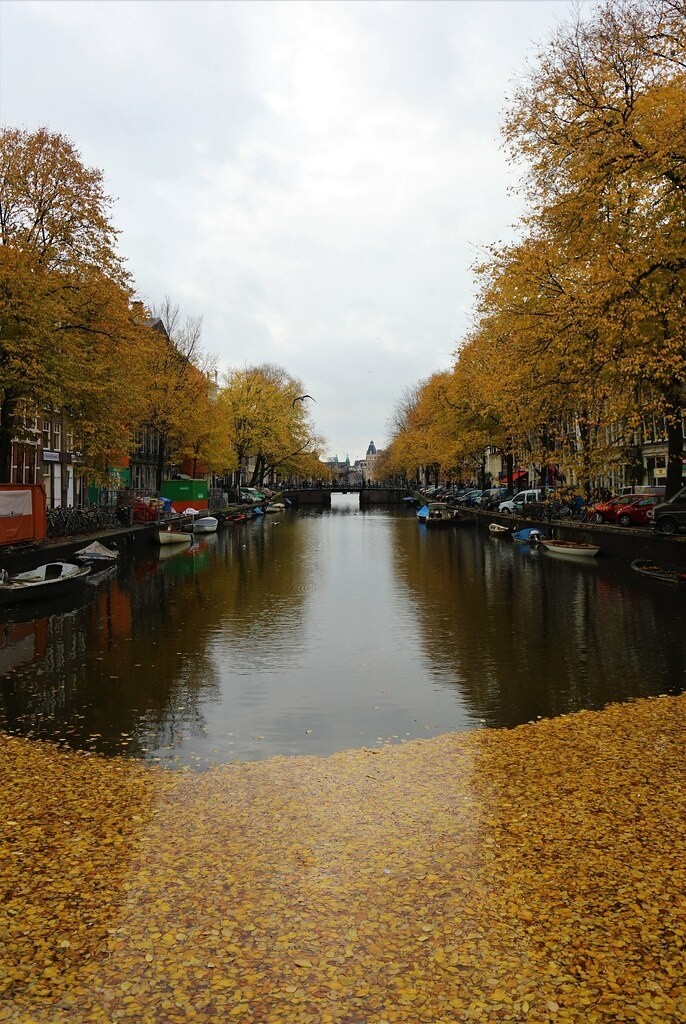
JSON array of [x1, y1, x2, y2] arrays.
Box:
[[503, 472, 528, 483]]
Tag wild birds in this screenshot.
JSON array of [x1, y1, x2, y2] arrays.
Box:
[[291, 394, 318, 410], [270, 521, 281, 525]]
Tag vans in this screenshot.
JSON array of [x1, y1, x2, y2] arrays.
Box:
[[239, 487, 265, 502]]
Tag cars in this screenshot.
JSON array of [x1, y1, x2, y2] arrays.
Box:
[[254, 487, 272, 499], [615, 493, 665, 527], [588, 494, 650, 524], [649, 486, 686, 537], [420, 484, 516, 512], [544, 495, 591, 520], [498, 488, 555, 516]]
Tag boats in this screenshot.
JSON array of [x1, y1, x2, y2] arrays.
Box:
[[0, 561, 92, 607], [221, 502, 286, 528], [631, 558, 686, 586], [151, 521, 195, 546], [488, 522, 521, 537], [540, 540, 601, 556], [182, 516, 218, 534], [400, 496, 420, 507], [416, 505, 429, 522], [425, 502, 472, 528], [74, 540, 120, 574]]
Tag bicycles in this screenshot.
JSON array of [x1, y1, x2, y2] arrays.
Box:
[[46, 502, 121, 539]]
